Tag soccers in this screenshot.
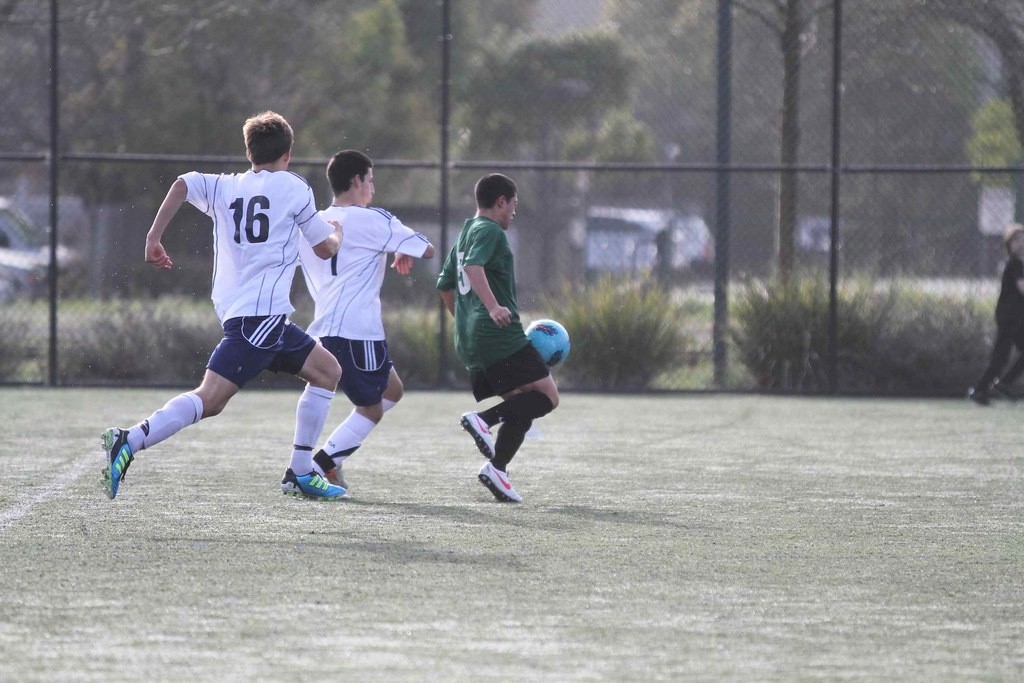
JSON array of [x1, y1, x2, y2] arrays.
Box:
[[525, 319, 571, 368]]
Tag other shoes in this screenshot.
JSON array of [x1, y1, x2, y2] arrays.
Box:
[[995, 382, 1017, 398], [970, 385, 989, 406]]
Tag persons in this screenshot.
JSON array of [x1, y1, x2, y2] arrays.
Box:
[[298, 150, 434, 489], [967, 223, 1024, 407], [437, 173, 560, 502], [102, 110, 349, 501]]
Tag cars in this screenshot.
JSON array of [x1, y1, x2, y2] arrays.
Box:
[[588, 206, 715, 287], [0, 199, 80, 297]]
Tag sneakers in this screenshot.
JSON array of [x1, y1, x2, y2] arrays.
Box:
[[459, 414, 495, 460], [312, 455, 347, 488], [282, 466, 347, 500], [102, 426, 133, 500], [478, 463, 520, 502]]
